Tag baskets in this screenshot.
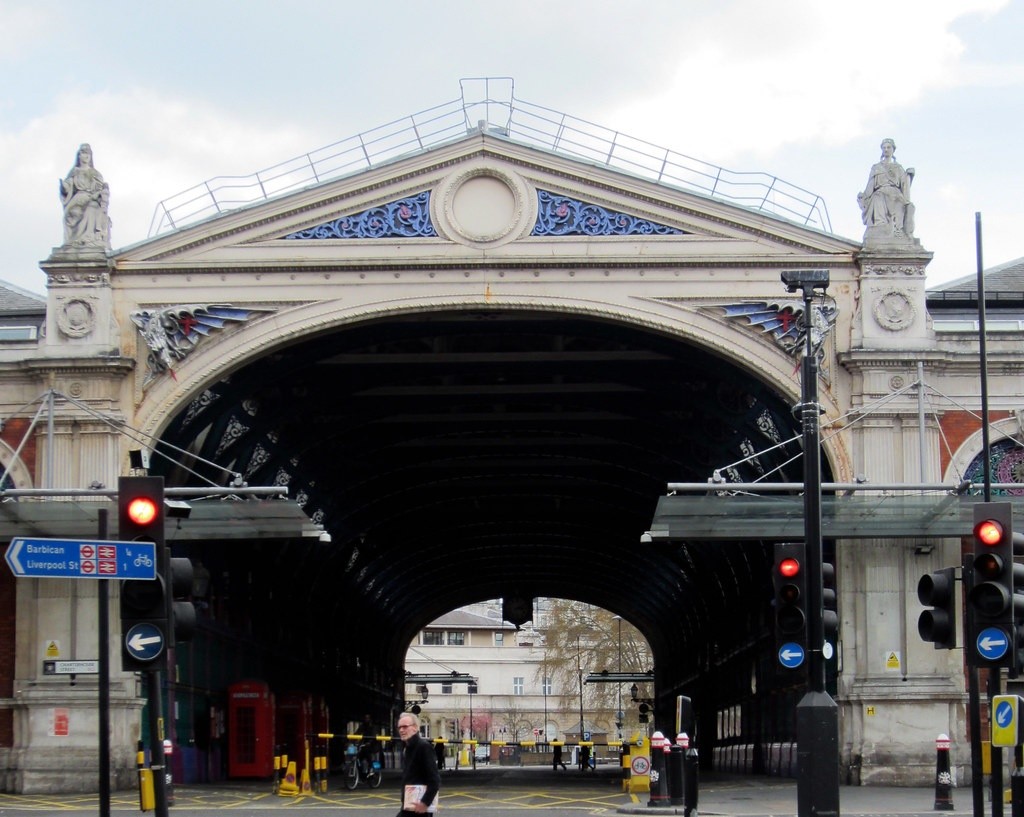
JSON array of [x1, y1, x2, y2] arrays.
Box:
[[344, 745, 358, 755]]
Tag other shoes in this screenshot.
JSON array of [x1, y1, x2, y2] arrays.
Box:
[[370, 766, 375, 776]]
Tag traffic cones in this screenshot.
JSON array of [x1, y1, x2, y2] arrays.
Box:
[[278, 762, 299, 798]]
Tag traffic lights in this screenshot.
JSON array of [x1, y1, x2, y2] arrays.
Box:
[[976, 506, 1017, 626], [918, 569, 958, 651], [773, 543, 808, 647], [118, 475, 171, 624]]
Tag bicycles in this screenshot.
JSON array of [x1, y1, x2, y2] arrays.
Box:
[[342, 741, 386, 791]]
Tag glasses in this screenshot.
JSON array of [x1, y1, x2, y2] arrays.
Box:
[[398, 725, 415, 730]]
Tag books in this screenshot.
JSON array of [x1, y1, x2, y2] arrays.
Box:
[[403, 785, 439, 813]]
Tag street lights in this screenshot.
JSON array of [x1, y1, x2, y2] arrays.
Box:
[[611, 615, 622, 741]]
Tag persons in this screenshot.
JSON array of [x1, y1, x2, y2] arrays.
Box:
[[352, 713, 375, 779], [59, 143, 110, 240], [581, 741, 594, 771], [857, 138, 910, 224], [552, 738, 567, 770], [429, 736, 446, 769], [397, 709, 440, 817]]
